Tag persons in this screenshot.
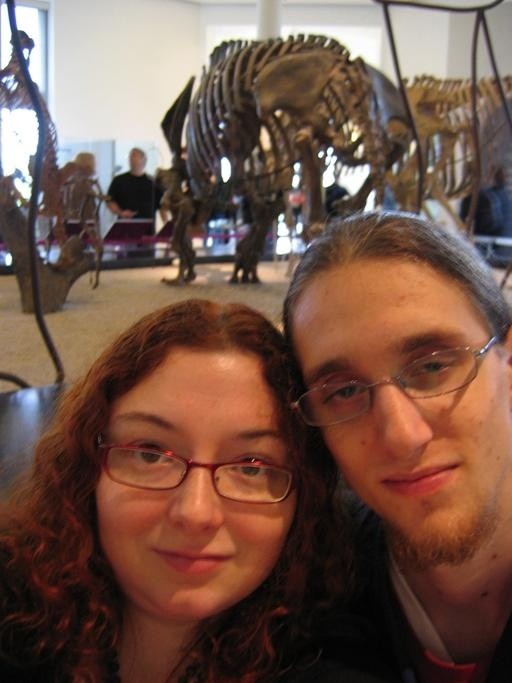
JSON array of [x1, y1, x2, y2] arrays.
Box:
[[322, 166, 351, 212], [161, 146, 190, 225], [103, 146, 165, 225], [280, 209, 512, 683], [62, 151, 106, 223], [457, 159, 502, 235], [488, 158, 512, 238], [0, 295, 360, 682]]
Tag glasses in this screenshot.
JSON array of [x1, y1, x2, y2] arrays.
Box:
[[290, 336, 509, 429], [97, 431, 296, 504]]
